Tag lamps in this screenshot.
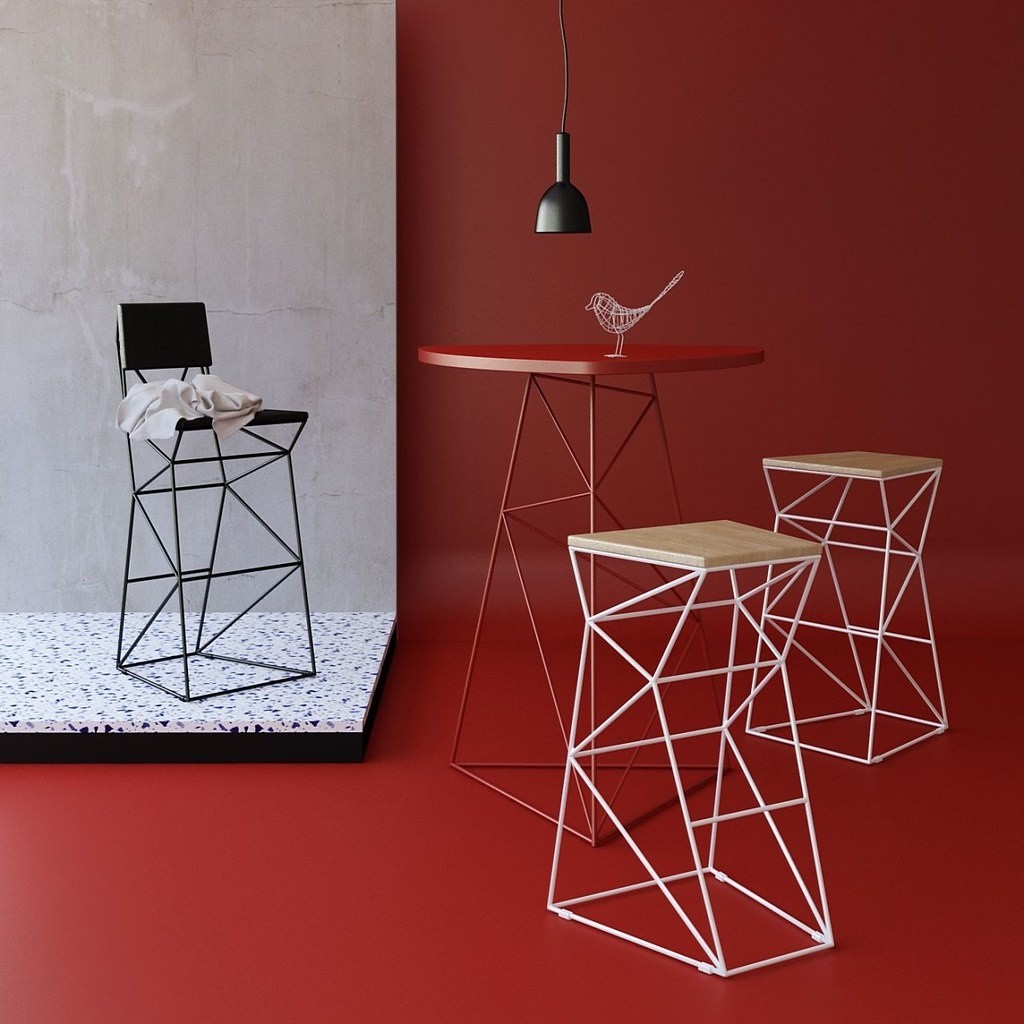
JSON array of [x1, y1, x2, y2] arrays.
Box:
[[535, 0, 592, 234]]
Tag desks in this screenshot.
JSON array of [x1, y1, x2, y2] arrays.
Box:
[[418, 343, 765, 844]]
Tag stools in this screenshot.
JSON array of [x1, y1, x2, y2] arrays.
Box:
[[547, 521, 835, 979], [744, 451, 948, 764], [116, 302, 316, 703]]
